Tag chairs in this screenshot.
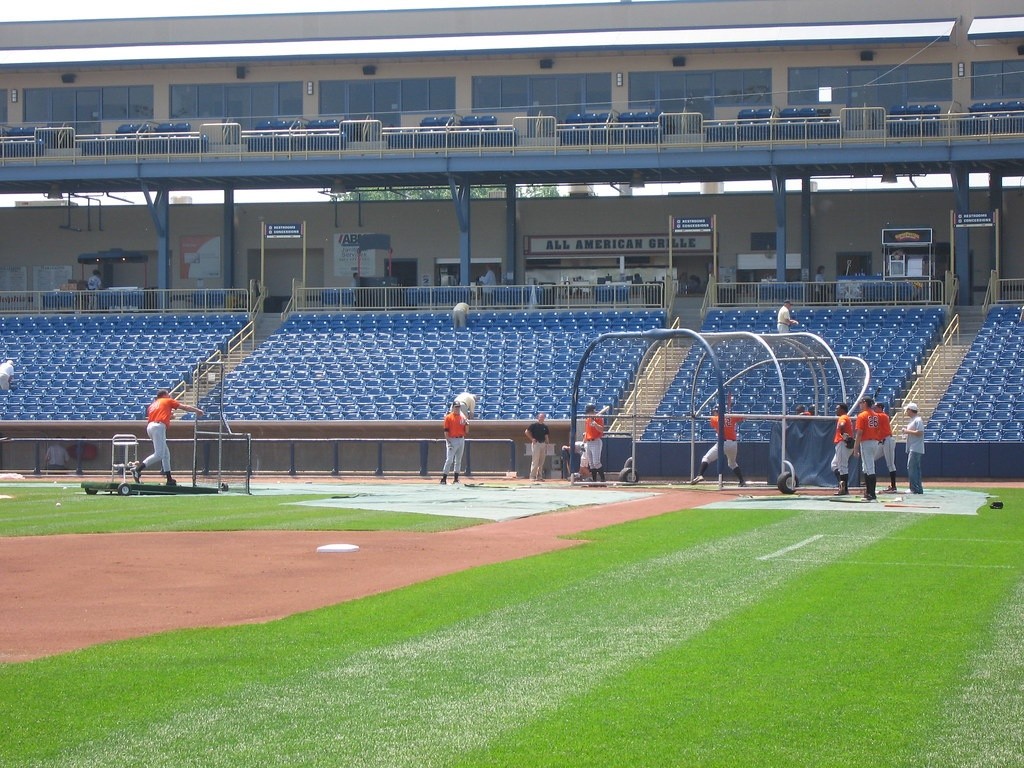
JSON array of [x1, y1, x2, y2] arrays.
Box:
[[0, 303, 1024, 443], [0, 102, 1023, 158]]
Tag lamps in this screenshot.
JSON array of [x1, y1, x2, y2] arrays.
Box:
[[616, 72, 622, 87], [630, 171, 645, 186], [11, 89, 18, 103], [958, 62, 965, 77], [881, 167, 897, 183], [46, 182, 63, 199], [307, 81, 314, 95], [329, 176, 346, 192]]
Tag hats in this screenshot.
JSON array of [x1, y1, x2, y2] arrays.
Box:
[[874, 402, 884, 408], [713, 404, 720, 410], [903, 403, 919, 410], [859, 396, 872, 403], [453, 401, 461, 408]]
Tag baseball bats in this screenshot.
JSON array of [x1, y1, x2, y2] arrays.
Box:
[[884, 504, 940, 508], [460, 410, 469, 425], [592, 406, 610, 419], [727, 391, 731, 415]]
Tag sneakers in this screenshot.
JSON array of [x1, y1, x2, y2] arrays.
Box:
[[738, 480, 746, 487], [166, 479, 176, 485], [691, 475, 705, 484], [130, 467, 141, 483]]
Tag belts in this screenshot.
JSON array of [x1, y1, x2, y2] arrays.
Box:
[[456, 436, 463, 438], [535, 441, 542, 443], [724, 440, 736, 441], [589, 439, 594, 441]]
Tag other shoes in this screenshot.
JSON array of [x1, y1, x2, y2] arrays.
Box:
[[864, 490, 876, 500], [904, 490, 913, 494], [453, 480, 460, 484], [440, 479, 446, 484], [883, 486, 897, 493], [834, 491, 849, 495]]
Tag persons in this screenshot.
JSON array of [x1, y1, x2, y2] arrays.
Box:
[[815, 266, 830, 306], [0, 360, 14, 390], [562, 442, 594, 482], [902, 403, 925, 494], [583, 403, 607, 487], [351, 273, 360, 311], [795, 404, 819, 416], [873, 402, 897, 493], [480, 265, 500, 310], [131, 389, 204, 485], [831, 404, 854, 495], [453, 303, 469, 328], [691, 405, 747, 486], [525, 414, 550, 481], [854, 396, 880, 500], [88, 270, 103, 313], [441, 402, 470, 485], [777, 300, 798, 334]]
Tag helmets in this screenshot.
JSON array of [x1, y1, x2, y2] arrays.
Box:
[[585, 403, 596, 411]]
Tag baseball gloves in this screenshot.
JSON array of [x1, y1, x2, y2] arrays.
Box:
[[844, 436, 856, 448], [990, 501, 1003, 509]]
